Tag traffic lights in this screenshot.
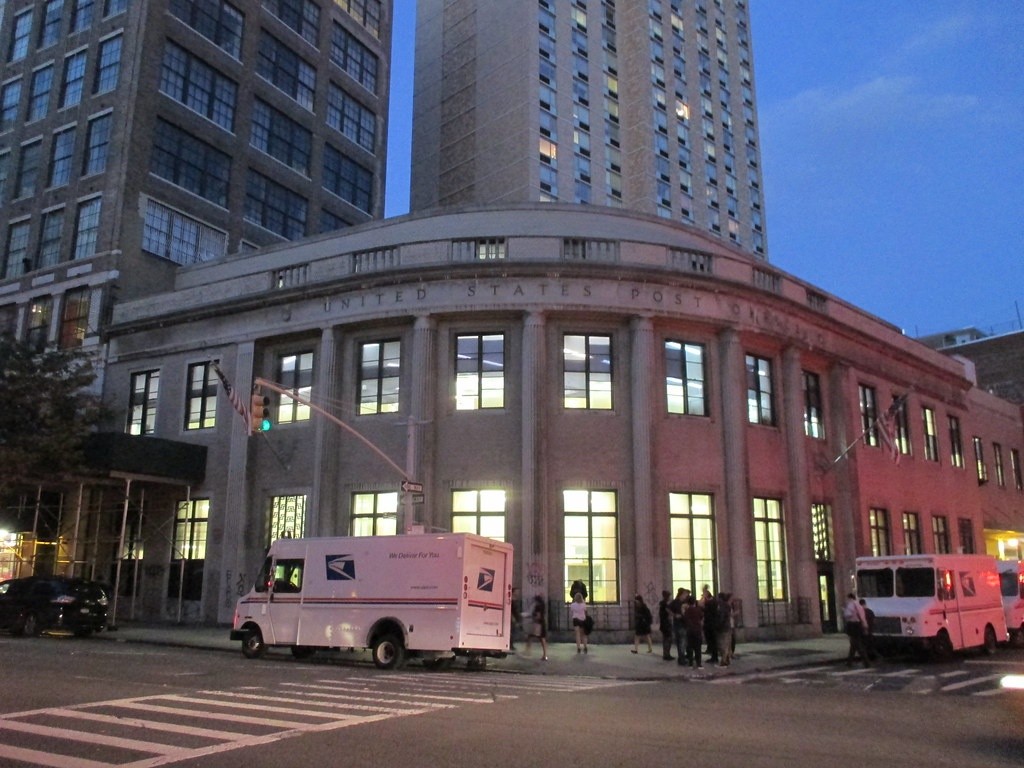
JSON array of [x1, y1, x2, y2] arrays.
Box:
[[250, 391, 271, 435]]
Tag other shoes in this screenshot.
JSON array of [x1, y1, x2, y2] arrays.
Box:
[[690, 666, 693, 669], [698, 666, 704, 669], [577, 648, 581, 654], [663, 656, 675, 660], [631, 650, 637, 653], [541, 657, 548, 661], [647, 648, 652, 653], [714, 664, 727, 668], [678, 661, 687, 665], [707, 659, 718, 663], [584, 647, 587, 654]]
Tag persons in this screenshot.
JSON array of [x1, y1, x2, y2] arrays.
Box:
[[714, 591, 732, 668], [659, 590, 676, 660], [670, 585, 736, 663], [682, 597, 705, 669], [858, 598, 877, 662], [571, 592, 587, 654], [510, 587, 522, 650], [521, 595, 548, 660], [631, 594, 653, 653], [844, 593, 874, 668]]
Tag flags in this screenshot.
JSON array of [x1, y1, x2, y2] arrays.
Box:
[[212, 367, 251, 435], [875, 398, 906, 467]]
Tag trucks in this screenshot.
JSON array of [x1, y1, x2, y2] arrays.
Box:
[[853, 554, 1010, 663], [234, 532, 515, 669]]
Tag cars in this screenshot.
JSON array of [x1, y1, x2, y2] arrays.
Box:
[[0, 577, 111, 639]]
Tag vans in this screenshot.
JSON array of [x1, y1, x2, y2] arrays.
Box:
[[996, 559, 1024, 641]]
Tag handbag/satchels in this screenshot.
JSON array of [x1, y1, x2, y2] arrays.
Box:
[[527, 623, 541, 636], [584, 616, 594, 635]]
